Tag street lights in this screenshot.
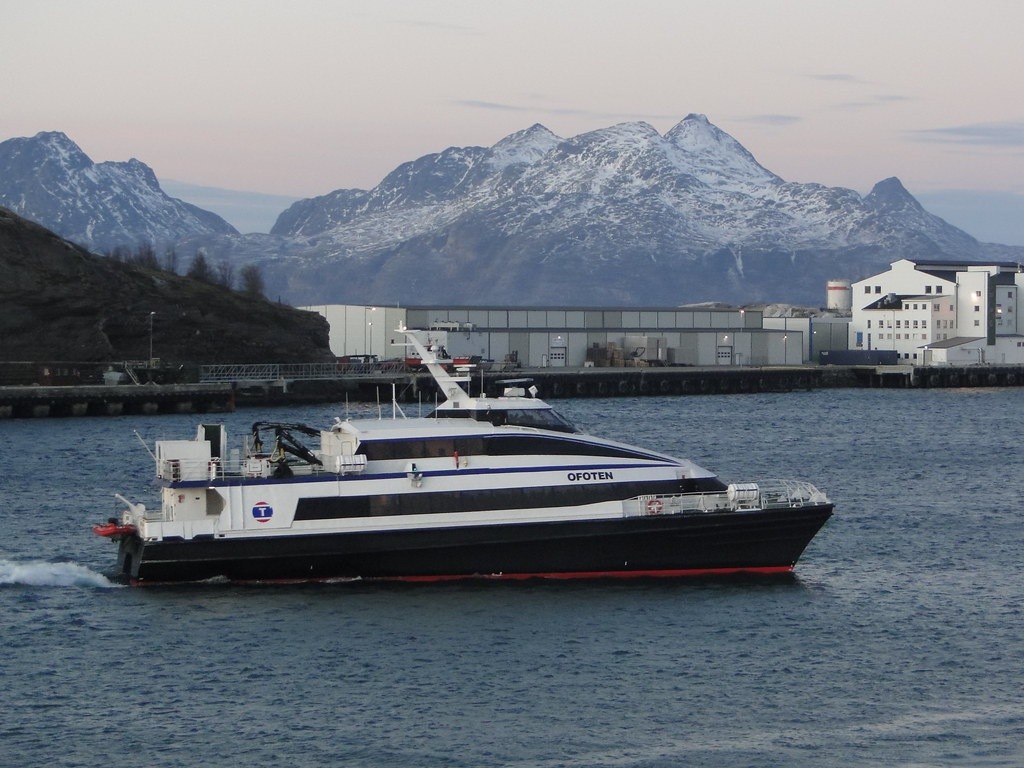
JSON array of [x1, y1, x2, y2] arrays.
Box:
[[364, 307, 376, 355], [149, 312, 155, 383], [369, 322, 373, 355]]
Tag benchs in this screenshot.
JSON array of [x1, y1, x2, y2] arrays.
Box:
[[429, 322, 460, 331]]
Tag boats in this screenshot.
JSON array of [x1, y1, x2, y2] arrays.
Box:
[[92, 384, 837, 588], [393, 319, 592, 437], [405, 332, 495, 372]]
